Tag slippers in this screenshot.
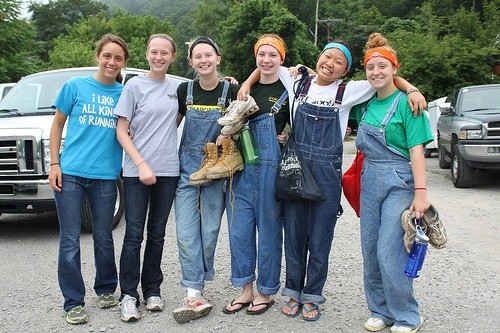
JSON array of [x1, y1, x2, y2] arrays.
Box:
[[364, 315, 386, 331], [246, 299, 275, 315], [281, 301, 301, 318], [302, 303, 321, 321], [391, 314, 425, 333], [222, 298, 251, 314]]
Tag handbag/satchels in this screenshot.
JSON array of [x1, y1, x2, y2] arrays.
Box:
[[275, 134, 328, 205], [341, 151, 364, 217]]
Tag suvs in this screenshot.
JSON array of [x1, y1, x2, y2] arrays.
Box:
[[437, 83, 500, 188]]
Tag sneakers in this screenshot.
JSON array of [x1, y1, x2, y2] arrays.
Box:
[[221, 120, 244, 135], [66, 305, 88, 325], [97, 291, 117, 309], [118, 294, 140, 322], [172, 295, 213, 325], [206, 137, 245, 226], [422, 204, 448, 250], [401, 208, 427, 254], [142, 295, 163, 311], [189, 141, 222, 214], [218, 94, 258, 126]]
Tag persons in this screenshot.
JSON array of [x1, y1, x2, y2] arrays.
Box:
[[349, 33, 433, 333], [236, 40, 427, 321], [222, 33, 290, 314], [49, 32, 128, 324], [172, 35, 241, 324], [114, 33, 239, 323]]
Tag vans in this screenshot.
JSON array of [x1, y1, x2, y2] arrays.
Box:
[[0, 66, 194, 233], [423, 95, 449, 158]]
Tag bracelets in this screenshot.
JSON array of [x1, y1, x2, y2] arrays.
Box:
[[50, 162, 59, 166], [135, 161, 144, 167], [414, 188, 427, 190], [407, 88, 420, 94]]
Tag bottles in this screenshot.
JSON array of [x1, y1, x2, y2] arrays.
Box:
[[240, 120, 259, 165], [405, 224, 430, 279]]
